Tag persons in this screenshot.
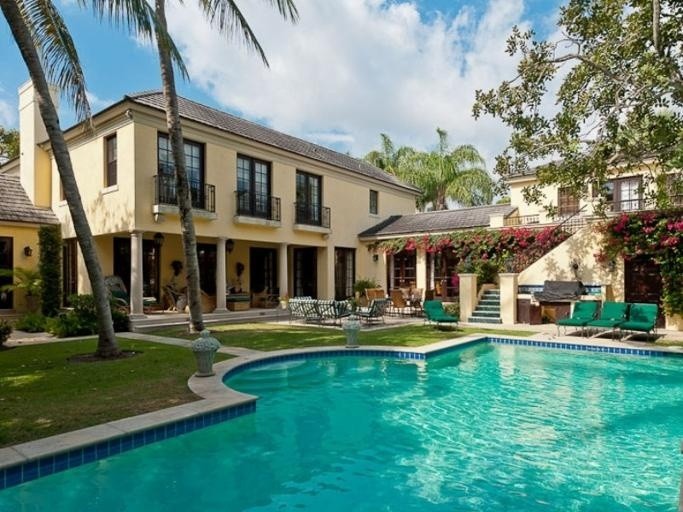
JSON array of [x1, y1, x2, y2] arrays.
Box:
[[161, 259, 185, 311]]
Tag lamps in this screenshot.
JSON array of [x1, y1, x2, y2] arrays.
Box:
[[372, 254, 378, 262], [24, 246, 33, 256], [153, 233, 164, 248], [226, 239, 234, 254]]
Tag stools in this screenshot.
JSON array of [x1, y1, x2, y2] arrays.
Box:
[[226, 296, 251, 311]]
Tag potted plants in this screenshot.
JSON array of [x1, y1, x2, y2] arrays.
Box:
[[0, 261, 49, 312]]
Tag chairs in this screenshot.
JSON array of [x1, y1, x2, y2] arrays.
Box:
[[337, 287, 422, 328], [184, 288, 216, 313], [255, 287, 279, 307], [423, 299, 459, 333], [557, 301, 659, 343], [162, 286, 187, 312], [110, 287, 165, 315]]
